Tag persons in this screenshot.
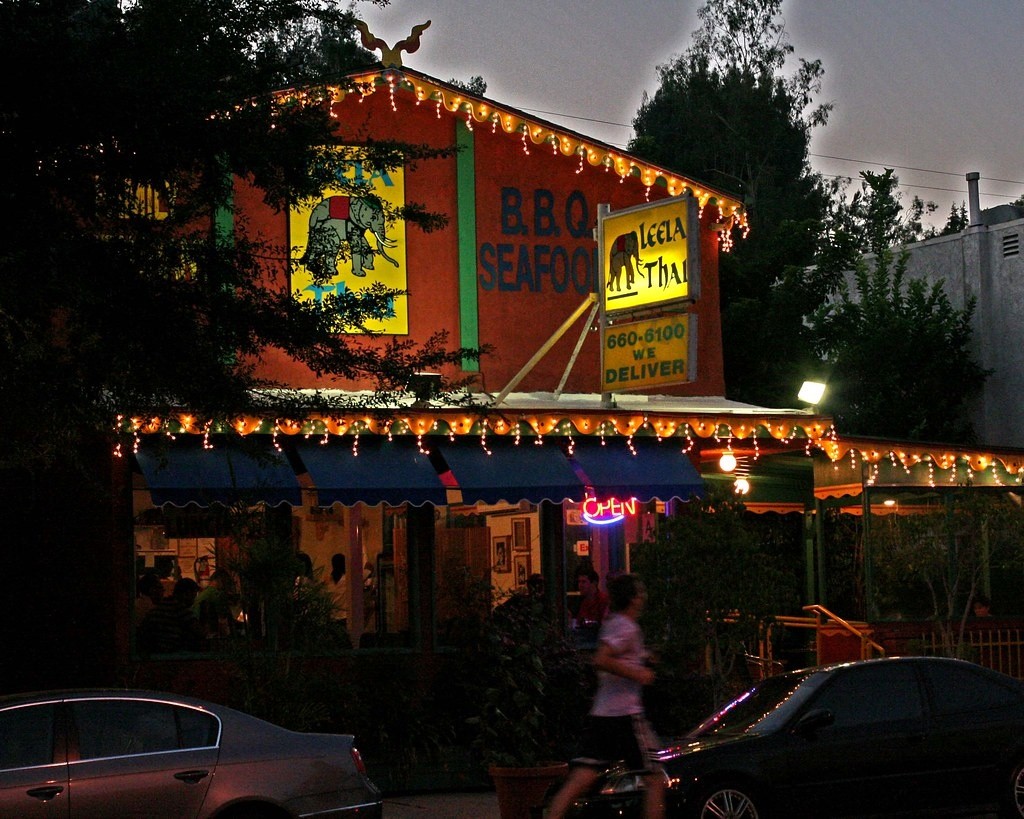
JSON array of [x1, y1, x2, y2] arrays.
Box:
[[575, 569, 608, 620], [136, 558, 246, 653], [527, 574, 545, 596], [973, 595, 992, 616], [496, 543, 505, 565], [322, 553, 348, 625], [295, 553, 316, 587], [547, 574, 665, 819]]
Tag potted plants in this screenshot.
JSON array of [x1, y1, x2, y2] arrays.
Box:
[[463, 632, 568, 819]]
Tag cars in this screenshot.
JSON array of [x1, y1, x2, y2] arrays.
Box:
[[538, 657, 1024, 819], [0, 688, 382, 819]]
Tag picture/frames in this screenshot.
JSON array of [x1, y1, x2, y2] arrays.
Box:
[[513, 555, 531, 589], [511, 518, 531, 552], [492, 536, 512, 573]]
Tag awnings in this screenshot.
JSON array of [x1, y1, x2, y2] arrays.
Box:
[[563, 437, 705, 503], [430, 434, 588, 507], [289, 437, 448, 508], [128, 435, 304, 507]]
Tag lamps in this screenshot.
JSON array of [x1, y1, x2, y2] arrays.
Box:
[[404, 372, 442, 402]]
